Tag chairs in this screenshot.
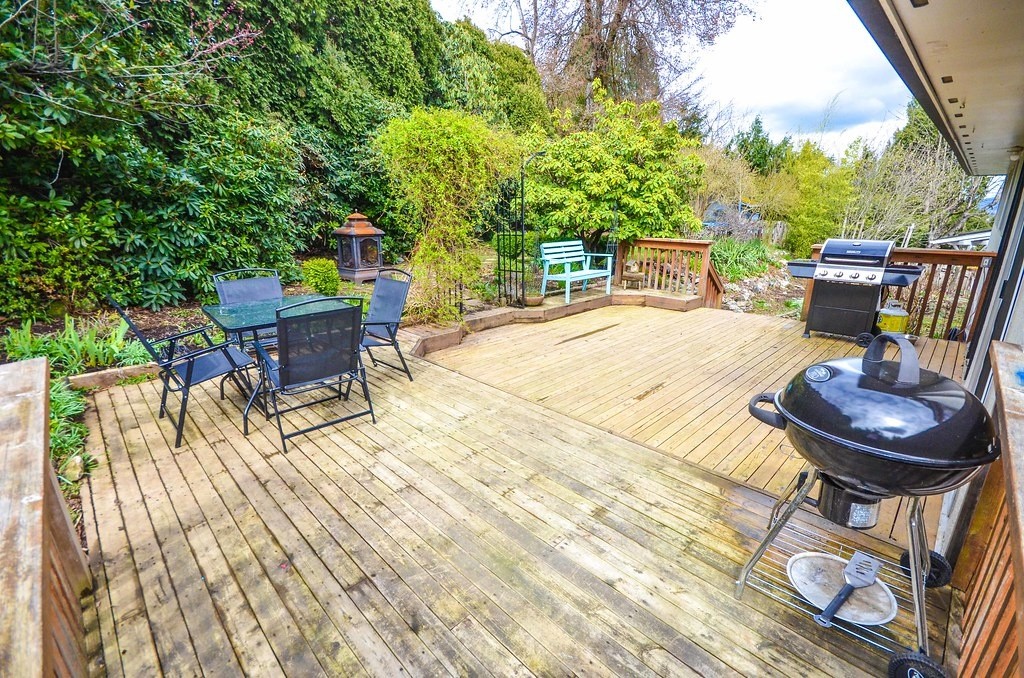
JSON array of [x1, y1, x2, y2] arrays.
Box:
[[251, 296, 377, 454], [343, 268, 414, 402], [212, 267, 285, 381], [107, 294, 272, 448]]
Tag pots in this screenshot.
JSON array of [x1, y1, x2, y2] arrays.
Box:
[[748, 333, 1000, 494]]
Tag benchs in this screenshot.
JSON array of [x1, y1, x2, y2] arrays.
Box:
[[540, 240, 613, 304]]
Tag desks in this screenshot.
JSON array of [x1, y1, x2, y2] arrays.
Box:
[[202, 294, 369, 436]]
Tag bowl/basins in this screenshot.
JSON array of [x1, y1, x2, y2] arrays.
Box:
[[904, 334, 919, 345]]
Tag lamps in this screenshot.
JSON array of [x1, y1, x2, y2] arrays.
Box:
[[1006, 146, 1023, 161]]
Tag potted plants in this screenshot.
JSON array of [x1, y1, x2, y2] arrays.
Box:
[[520, 287, 544, 306]]
